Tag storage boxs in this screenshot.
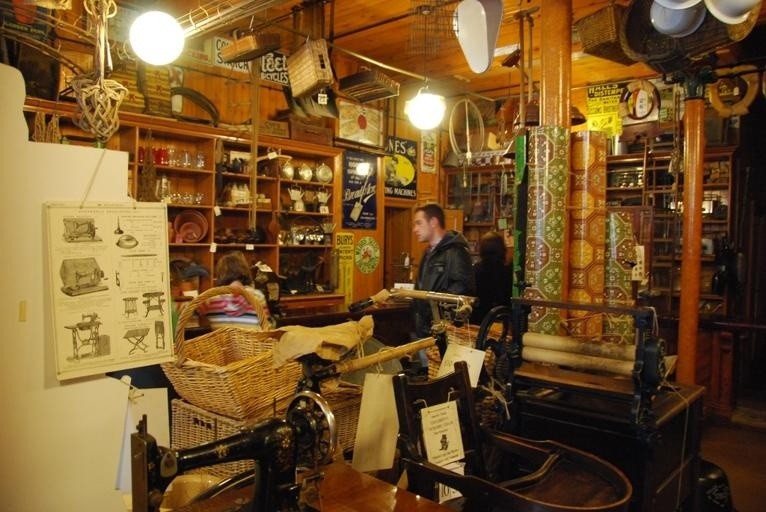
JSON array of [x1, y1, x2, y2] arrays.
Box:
[[219, 30, 336, 149]]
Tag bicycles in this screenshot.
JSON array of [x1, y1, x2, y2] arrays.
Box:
[[308, 285, 480, 398]]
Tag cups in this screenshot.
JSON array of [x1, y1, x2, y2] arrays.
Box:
[[138, 146, 208, 171], [157, 174, 205, 205]]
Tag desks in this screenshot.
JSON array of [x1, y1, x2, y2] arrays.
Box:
[[514, 355, 707, 512]]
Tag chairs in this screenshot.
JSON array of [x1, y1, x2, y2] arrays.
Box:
[[390, 359, 634, 511]]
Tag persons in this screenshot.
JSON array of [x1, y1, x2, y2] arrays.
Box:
[[471, 231, 511, 327], [198, 253, 271, 331], [411, 203, 474, 331]]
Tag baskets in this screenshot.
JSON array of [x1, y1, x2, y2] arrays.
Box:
[[282, 36, 339, 103], [153, 279, 336, 422], [162, 375, 365, 486], [422, 313, 519, 436], [217, 25, 285, 66]]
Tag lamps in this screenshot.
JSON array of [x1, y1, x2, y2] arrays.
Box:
[[404, 6, 448, 133]]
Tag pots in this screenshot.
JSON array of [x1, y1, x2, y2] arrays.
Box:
[[280, 160, 333, 183]]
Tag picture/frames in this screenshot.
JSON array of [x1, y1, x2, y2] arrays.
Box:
[[44, 200, 178, 383]]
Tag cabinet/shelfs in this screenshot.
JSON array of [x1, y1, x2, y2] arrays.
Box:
[[23, 98, 347, 303], [445, 162, 515, 268], [606, 145, 737, 330]]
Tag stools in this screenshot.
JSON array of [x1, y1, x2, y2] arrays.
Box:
[[688, 458, 734, 512]]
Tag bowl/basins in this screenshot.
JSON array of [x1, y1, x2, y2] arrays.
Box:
[[174, 210, 210, 242], [648, 1, 759, 40]]
